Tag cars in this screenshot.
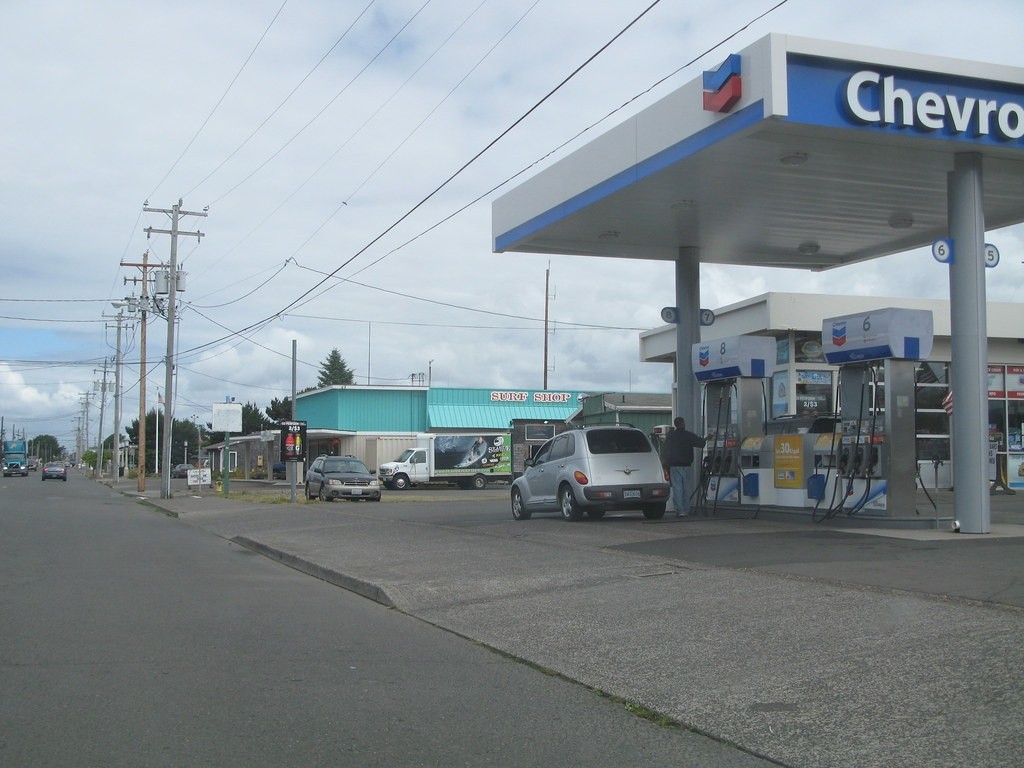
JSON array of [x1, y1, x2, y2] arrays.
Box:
[[42, 463, 69, 481], [27, 459, 38, 471], [172, 464, 195, 479]]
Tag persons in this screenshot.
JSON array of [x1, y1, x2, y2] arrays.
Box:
[[662, 418, 706, 516]]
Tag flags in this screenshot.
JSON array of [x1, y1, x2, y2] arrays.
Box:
[[942, 391, 954, 417], [915, 362, 938, 393]]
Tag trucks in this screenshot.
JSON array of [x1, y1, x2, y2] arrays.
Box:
[[2, 440, 28, 478], [377, 434, 512, 490]]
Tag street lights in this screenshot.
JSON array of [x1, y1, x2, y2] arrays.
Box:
[[111, 300, 176, 498]]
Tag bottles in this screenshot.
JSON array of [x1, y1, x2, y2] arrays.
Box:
[[285, 433, 295, 457], [294, 434, 303, 455]]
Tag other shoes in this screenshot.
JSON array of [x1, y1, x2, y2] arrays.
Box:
[[678, 512, 687, 516]]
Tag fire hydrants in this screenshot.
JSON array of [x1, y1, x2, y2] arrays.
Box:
[[214, 476, 223, 492]]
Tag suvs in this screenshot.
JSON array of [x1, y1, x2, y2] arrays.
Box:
[[304, 455, 381, 502], [510, 423, 671, 522]]
